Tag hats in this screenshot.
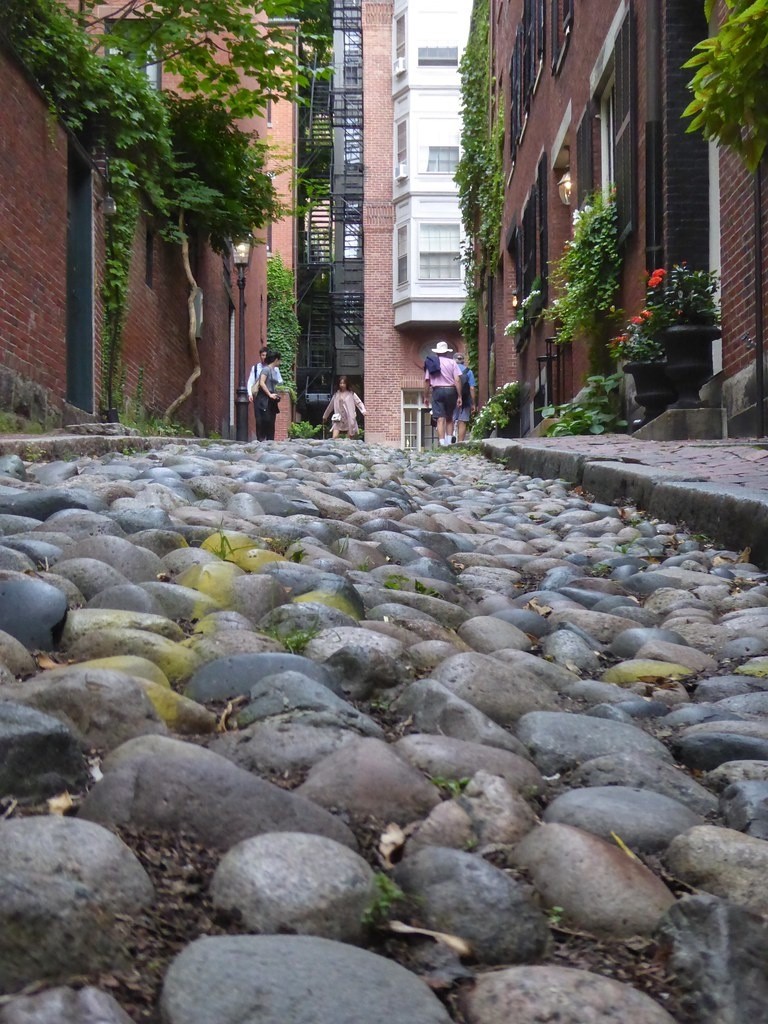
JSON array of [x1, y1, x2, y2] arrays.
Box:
[[431, 341, 453, 353]]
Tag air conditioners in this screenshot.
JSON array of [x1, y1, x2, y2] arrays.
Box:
[[393, 57, 406, 75], [394, 164, 407, 180]]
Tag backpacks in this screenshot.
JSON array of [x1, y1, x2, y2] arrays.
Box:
[[459, 367, 470, 408]]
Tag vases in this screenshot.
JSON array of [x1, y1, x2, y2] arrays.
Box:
[[623, 363, 678, 427], [513, 328, 527, 349], [526, 298, 542, 324], [661, 326, 722, 410], [490, 429, 515, 437]]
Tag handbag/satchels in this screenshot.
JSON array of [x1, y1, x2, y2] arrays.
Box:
[[251, 380, 260, 397], [331, 413, 342, 421]]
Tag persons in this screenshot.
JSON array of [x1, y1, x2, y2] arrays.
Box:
[[248, 347, 284, 442], [450, 353, 476, 443], [323, 376, 368, 440], [424, 341, 462, 447]]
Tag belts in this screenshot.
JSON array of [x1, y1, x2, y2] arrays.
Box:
[[435, 386, 455, 388]]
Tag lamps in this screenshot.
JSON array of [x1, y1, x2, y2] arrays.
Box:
[[513, 289, 518, 307], [557, 144, 572, 205], [95, 191, 116, 216]]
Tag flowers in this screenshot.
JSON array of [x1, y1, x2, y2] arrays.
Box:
[[471, 381, 520, 439], [504, 290, 542, 337], [606, 261, 721, 362]]
